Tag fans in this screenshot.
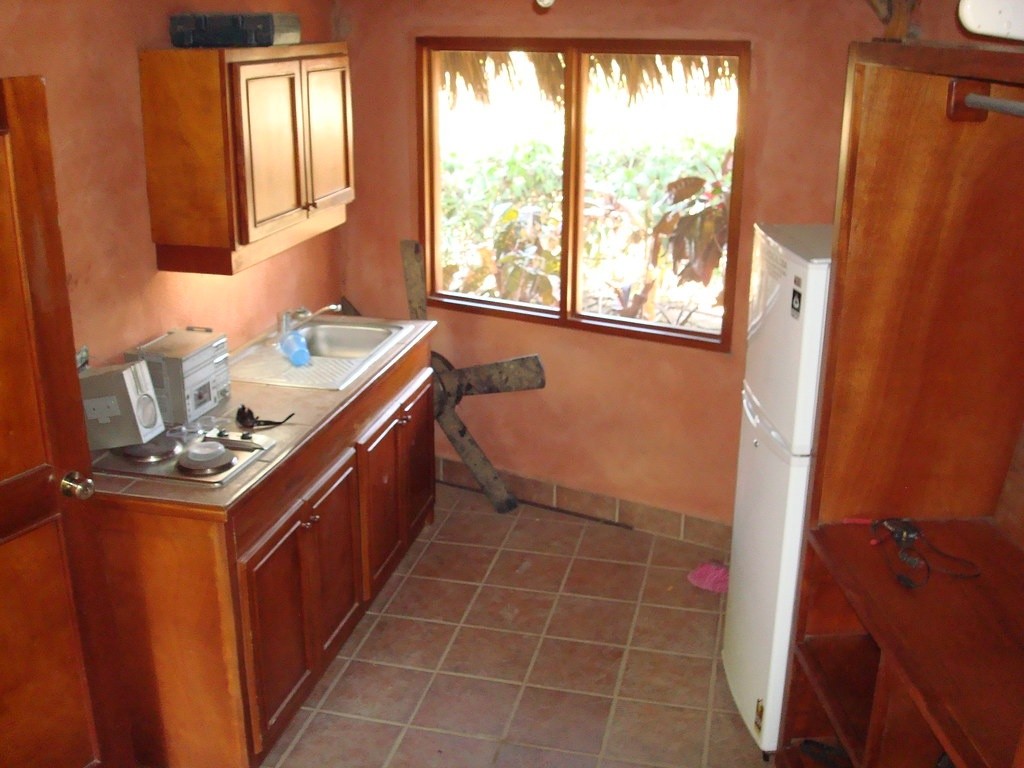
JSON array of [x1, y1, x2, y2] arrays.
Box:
[[336, 239, 546, 515]]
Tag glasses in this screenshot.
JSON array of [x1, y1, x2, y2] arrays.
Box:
[[236, 405, 294, 429]]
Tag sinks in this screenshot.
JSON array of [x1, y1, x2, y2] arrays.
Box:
[[275, 325, 392, 360]]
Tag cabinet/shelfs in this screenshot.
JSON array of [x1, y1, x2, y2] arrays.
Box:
[[775, 41, 1024, 768], [135, 37, 357, 276], [94, 410, 367, 768], [348, 334, 438, 612]]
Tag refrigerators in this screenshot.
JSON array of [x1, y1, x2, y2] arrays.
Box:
[[721, 221, 834, 752]]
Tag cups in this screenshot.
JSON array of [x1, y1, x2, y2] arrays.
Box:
[[281, 333, 311, 367]]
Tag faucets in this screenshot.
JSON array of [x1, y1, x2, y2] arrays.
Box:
[[266, 304, 343, 341]]
